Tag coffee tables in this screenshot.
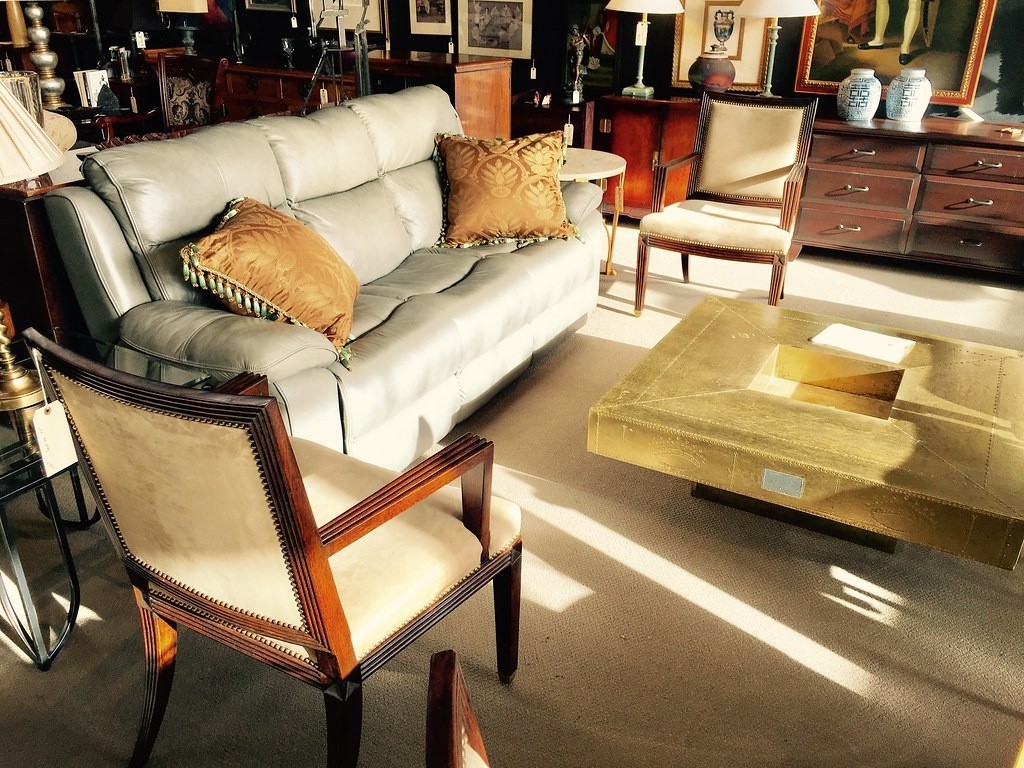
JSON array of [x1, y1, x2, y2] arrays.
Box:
[[587, 293, 1024, 572]]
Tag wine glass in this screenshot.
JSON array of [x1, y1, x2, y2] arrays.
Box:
[[282, 38, 297, 70]]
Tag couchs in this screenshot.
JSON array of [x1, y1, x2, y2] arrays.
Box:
[[45, 85, 602, 473]]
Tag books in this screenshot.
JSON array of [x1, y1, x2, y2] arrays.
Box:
[[73, 69, 110, 108]]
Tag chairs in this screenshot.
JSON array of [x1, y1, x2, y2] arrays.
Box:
[[96, 53, 228, 143], [21, 327, 524, 768], [633, 94, 819, 317]]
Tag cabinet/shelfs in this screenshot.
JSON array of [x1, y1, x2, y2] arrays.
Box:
[[364, 48, 512, 142], [202, 58, 359, 122], [508, 91, 1023, 278]]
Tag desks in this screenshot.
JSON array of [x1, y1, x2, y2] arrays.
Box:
[[560, 148, 627, 277], [0, 140, 102, 350], [0, 327, 269, 671]]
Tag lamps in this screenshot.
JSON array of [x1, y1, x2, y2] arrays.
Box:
[[733, 0, 822, 95], [159, 0, 209, 56], [604, 0, 685, 96], [0, 80, 66, 411]]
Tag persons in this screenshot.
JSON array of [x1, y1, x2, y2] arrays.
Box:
[[569, 24, 590, 86]]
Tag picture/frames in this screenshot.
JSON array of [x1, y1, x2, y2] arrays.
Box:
[[409, 0, 452, 35], [794, 0, 996, 107], [458, 0, 533, 60], [561, 0, 623, 90], [245, 0, 297, 13], [672, 0, 772, 93], [309, 0, 383, 34]]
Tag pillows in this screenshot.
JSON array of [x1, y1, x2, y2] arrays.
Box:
[[180, 197, 360, 370], [432, 131, 584, 254]]
